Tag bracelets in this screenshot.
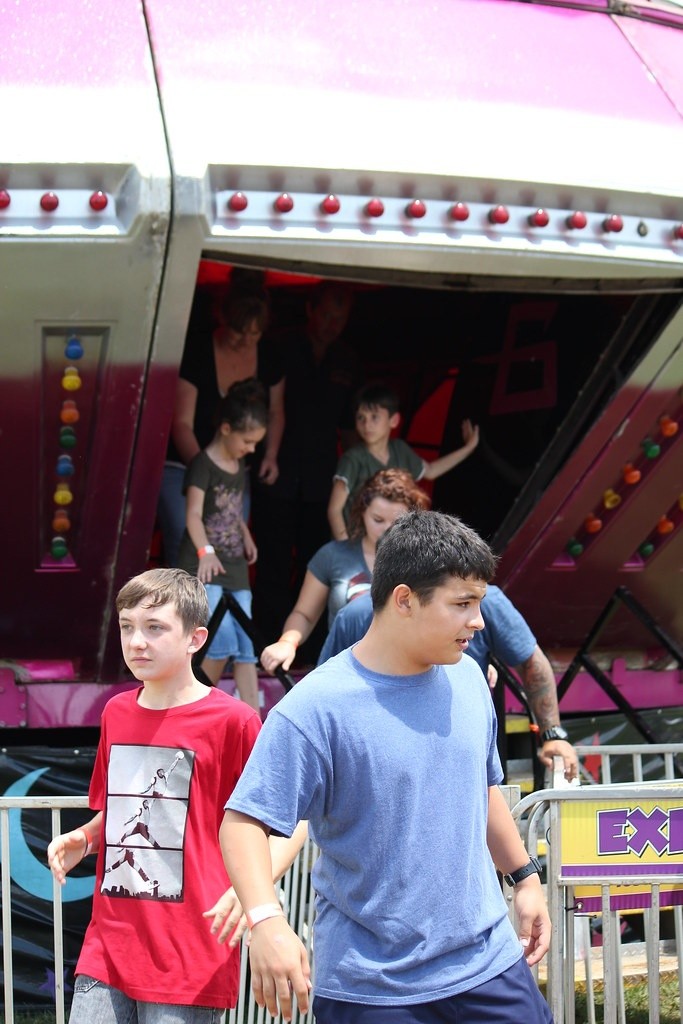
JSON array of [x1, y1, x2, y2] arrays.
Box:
[[246, 903, 285, 931], [77, 826, 92, 859], [197, 546, 214, 560]]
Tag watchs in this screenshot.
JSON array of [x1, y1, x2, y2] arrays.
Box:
[[504, 857, 543, 888], [540, 727, 567, 747]]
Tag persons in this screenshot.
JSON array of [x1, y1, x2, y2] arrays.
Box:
[[319, 584, 579, 785], [220, 510, 579, 1024], [260, 468, 430, 674], [182, 376, 271, 722], [327, 385, 480, 547], [156, 287, 285, 569], [48, 568, 309, 1024], [262, 279, 354, 669]]
